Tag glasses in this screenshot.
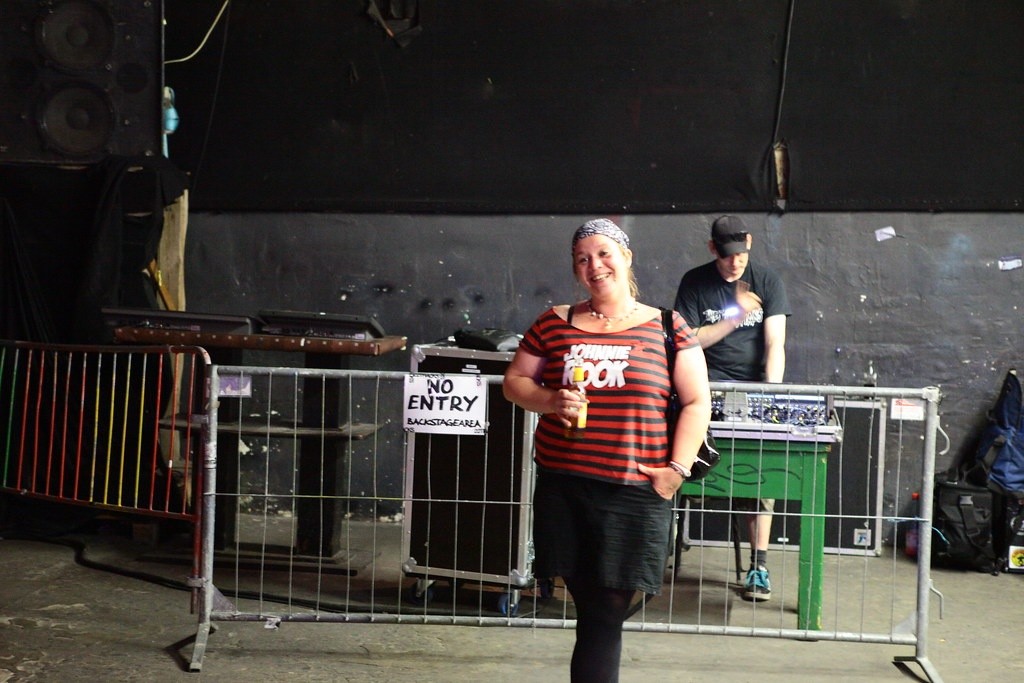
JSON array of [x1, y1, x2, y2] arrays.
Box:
[[719, 233, 746, 244]]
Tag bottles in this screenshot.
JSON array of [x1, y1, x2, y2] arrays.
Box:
[[906, 492, 920, 556], [570, 358, 586, 429]]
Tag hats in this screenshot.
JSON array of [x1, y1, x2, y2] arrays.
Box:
[[712, 215, 750, 259]]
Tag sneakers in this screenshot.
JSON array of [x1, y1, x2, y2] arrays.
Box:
[[744, 562, 771, 600]]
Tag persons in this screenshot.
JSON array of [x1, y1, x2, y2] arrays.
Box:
[[673, 216, 793, 601], [503, 218, 711, 683]]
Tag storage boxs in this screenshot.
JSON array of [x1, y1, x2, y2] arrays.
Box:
[[401, 344, 554, 617]]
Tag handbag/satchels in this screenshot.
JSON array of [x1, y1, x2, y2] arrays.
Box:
[[931, 368, 1024, 575], [658, 306, 721, 481]]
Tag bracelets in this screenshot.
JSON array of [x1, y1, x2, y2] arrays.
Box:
[[668, 460, 691, 477]]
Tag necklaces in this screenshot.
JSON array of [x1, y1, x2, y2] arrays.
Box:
[[586, 297, 638, 330]]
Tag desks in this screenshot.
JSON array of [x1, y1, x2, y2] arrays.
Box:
[[679, 439, 831, 642], [115, 328, 408, 580]]
